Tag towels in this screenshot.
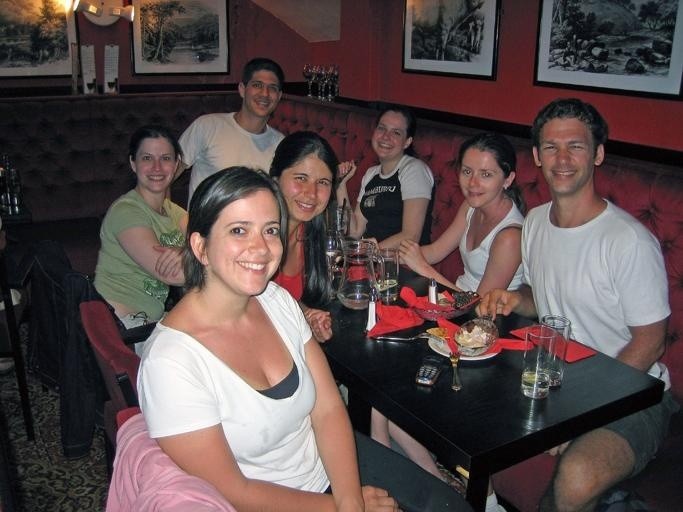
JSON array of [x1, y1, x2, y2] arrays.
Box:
[[400, 286, 481, 313], [367, 301, 425, 337], [436, 315, 534, 356], [509, 325, 597, 363], [339, 266, 380, 283]]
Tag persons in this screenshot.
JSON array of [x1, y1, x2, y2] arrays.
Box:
[[394, 131, 525, 304], [171, 57, 285, 214], [476, 97, 683, 511], [136, 165, 475, 512], [94, 125, 195, 344], [336, 105, 434, 255], [268, 131, 443, 483]]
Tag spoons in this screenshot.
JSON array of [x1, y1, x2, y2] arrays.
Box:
[[337, 198, 347, 234]]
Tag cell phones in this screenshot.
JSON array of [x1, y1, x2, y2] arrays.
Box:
[[412, 352, 445, 389]]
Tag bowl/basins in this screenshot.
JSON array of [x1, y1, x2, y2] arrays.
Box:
[[415, 291, 480, 321]]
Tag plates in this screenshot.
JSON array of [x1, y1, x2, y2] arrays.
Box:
[[428, 338, 502, 361]]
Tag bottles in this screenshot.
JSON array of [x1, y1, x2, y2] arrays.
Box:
[[366, 287, 377, 332], [428, 279, 437, 313]]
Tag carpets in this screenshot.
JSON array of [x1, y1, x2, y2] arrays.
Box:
[[0, 319, 110, 512]]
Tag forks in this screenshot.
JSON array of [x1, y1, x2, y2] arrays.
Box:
[[373, 332, 444, 344], [448, 352, 463, 392]]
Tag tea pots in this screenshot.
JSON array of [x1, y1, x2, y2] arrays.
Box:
[[337, 241, 387, 312]]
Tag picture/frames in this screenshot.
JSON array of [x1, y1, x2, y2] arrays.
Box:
[[128, 0, 231, 77], [401, 0, 502, 81], [533, 0, 683, 101], [0, 0, 83, 80]]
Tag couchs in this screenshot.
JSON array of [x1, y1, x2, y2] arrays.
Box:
[[5, 94, 683, 512]]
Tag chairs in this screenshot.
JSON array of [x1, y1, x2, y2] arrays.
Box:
[[0, 231, 34, 441], [80, 301, 157, 483]]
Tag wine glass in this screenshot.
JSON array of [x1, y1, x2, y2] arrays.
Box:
[[327, 64, 339, 104], [314, 66, 323, 100], [318, 67, 327, 100], [324, 229, 341, 281], [303, 63, 315, 98]]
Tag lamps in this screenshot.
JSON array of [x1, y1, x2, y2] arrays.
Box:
[[73, 0, 103, 17], [109, 5, 135, 22]]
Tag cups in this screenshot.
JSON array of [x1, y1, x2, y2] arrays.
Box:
[[537, 314, 571, 389], [326, 207, 351, 241], [520, 325, 558, 399], [378, 247, 400, 303]]
